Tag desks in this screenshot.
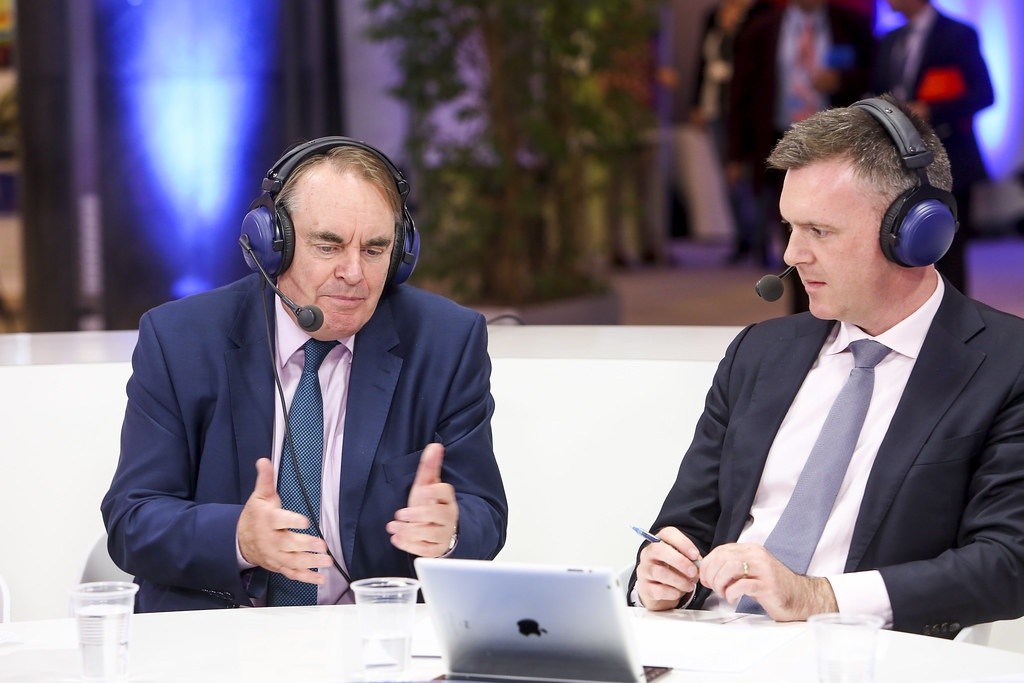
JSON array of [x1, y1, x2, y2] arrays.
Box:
[[0, 603, 1024, 683]]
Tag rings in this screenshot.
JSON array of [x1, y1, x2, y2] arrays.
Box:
[[743, 561, 749, 578]]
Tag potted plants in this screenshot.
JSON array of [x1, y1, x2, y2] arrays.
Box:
[[357, 0, 667, 326]]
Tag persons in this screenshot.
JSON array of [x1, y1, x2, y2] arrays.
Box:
[[627, 98, 1024, 640], [690, 0, 996, 315], [101, 145, 510, 616]]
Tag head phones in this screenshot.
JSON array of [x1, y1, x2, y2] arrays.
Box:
[[848, 98, 960, 268], [238, 134, 423, 292]]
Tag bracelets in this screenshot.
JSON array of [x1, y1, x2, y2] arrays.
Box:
[[449, 534, 458, 550]]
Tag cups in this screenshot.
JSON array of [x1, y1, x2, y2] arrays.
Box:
[[808, 612, 885, 683], [349, 575, 421, 682], [70, 580, 140, 682]]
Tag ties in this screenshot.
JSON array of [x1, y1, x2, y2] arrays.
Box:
[[889, 23, 914, 100], [264, 338, 342, 606], [790, 15, 819, 130], [736, 340, 894, 614]]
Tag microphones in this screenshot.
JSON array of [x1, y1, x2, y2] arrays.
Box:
[[249, 250, 325, 332], [754, 265, 794, 303]]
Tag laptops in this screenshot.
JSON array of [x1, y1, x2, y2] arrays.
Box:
[[413, 555, 676, 683]]
